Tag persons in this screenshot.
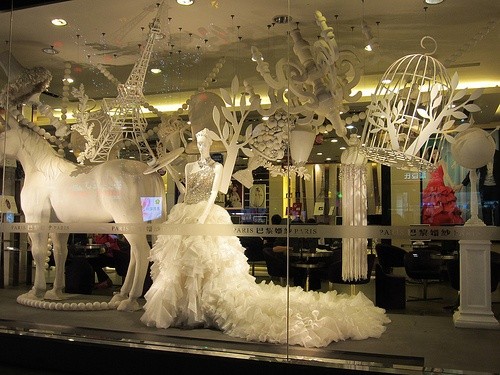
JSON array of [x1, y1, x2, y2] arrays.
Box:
[[230, 184, 242, 208], [421, 145, 465, 226], [91, 233, 131, 289], [254, 187, 262, 207], [140, 128, 392, 349], [282, 218, 304, 247], [263, 214, 282, 244], [455, 136, 500, 245], [303, 218, 317, 248]]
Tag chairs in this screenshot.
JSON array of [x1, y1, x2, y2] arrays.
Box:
[[240, 237, 290, 285], [375, 239, 459, 318]]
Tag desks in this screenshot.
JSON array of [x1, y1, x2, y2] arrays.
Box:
[[290, 263, 323, 291], [294, 251, 337, 263], [431, 253, 454, 295]]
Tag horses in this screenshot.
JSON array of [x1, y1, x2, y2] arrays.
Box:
[[0, 127, 167, 311]]
